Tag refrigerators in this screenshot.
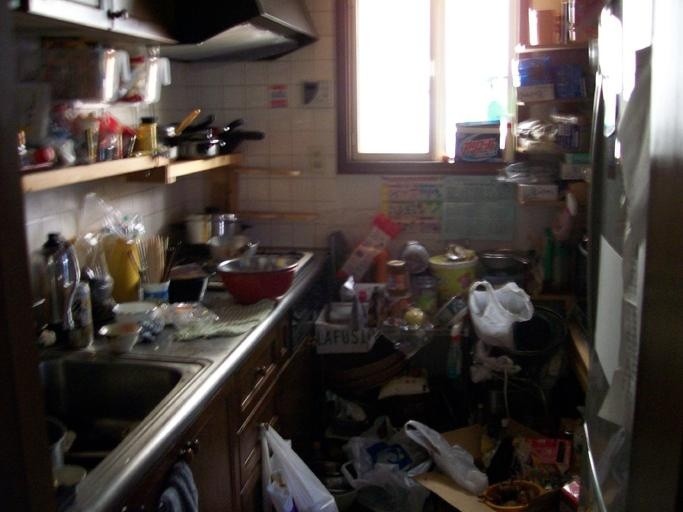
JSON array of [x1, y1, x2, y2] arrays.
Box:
[[576, 45, 652, 512]]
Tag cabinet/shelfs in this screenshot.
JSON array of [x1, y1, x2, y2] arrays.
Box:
[[230, 265, 335, 511], [516, 2, 591, 207], [106, 375, 236, 511]]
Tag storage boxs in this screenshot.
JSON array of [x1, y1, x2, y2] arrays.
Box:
[[411, 415, 557, 510]]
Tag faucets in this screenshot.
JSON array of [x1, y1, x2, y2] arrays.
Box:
[[29, 297, 51, 353]]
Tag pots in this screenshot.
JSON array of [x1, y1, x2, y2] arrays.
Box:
[[170, 206, 253, 243], [160, 113, 263, 161]]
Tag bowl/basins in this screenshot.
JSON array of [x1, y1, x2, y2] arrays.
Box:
[[216, 254, 298, 304], [476, 249, 524, 272], [480, 477, 542, 508]]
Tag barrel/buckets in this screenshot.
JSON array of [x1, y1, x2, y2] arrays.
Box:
[[427, 253, 477, 302]]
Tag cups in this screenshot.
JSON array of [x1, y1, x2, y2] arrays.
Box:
[[112, 300, 158, 336], [99, 324, 141, 355], [141, 279, 170, 304]]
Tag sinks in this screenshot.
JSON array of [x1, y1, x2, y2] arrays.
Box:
[[33, 349, 215, 512]]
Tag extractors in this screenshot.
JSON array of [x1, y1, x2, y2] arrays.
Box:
[[158, 0, 319, 63]]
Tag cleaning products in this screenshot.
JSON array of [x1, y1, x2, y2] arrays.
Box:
[[41, 232, 81, 343]]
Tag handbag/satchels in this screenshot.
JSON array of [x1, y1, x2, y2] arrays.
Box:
[[403, 420, 489, 492], [468, 280, 533, 349], [341, 415, 433, 512], [261, 422, 339, 512]]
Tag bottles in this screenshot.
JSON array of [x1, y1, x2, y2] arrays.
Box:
[[410, 275, 438, 319], [504, 122, 516, 164], [136, 117, 156, 152]]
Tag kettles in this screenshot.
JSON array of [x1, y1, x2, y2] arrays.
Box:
[[42, 231, 77, 338]]
